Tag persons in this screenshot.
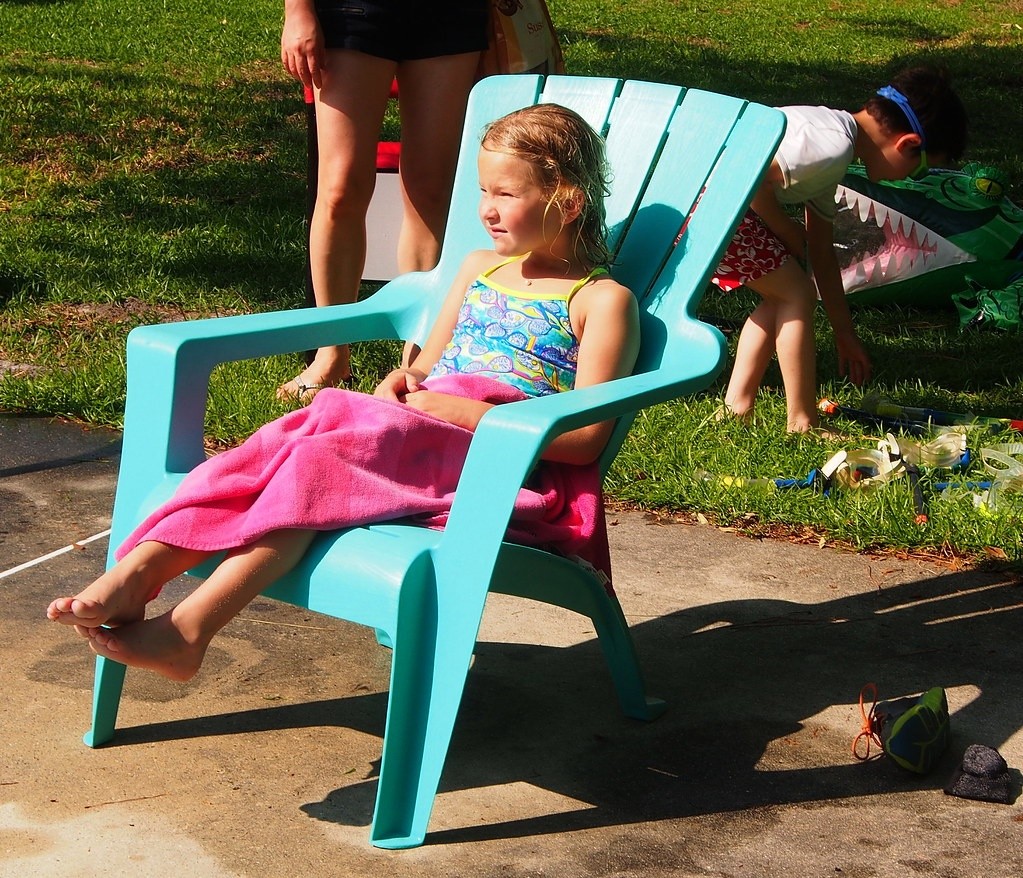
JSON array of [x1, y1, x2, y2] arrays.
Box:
[[672, 68, 968, 440], [277, 0, 491, 400], [44, 103, 640, 680]]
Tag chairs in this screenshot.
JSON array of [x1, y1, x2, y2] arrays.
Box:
[[82, 73, 786, 848]]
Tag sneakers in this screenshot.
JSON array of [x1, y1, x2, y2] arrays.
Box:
[[852, 682, 951, 776]]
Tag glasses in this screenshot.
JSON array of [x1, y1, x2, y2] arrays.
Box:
[[910, 150, 937, 181]]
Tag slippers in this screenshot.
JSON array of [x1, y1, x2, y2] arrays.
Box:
[[275, 374, 359, 409]]
